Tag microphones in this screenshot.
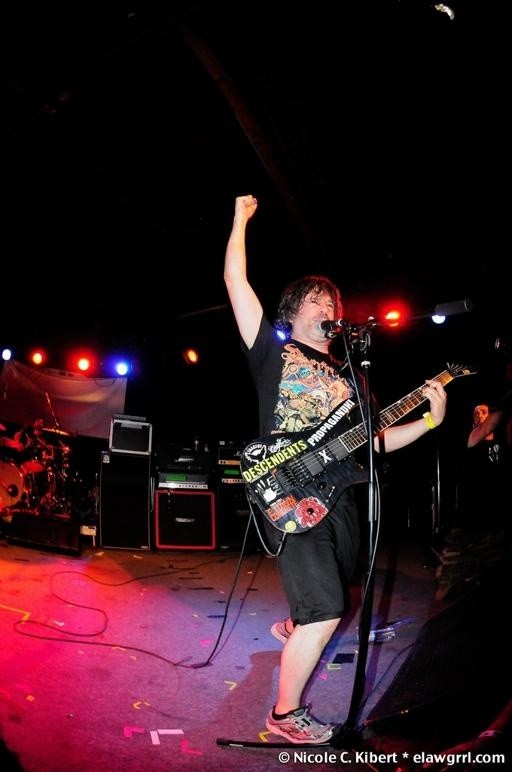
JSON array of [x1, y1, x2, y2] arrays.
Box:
[[315, 317, 348, 334]]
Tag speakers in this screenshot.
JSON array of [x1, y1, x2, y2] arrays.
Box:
[[98, 449, 151, 550], [155, 490, 217, 555]]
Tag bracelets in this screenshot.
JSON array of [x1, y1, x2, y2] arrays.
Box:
[[422, 412, 436, 431]]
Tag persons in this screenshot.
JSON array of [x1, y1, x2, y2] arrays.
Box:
[[19, 416, 46, 445], [463, 401, 511, 477], [219, 193, 452, 746]]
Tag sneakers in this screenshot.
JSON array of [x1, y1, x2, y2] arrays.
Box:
[[265, 706, 332, 744], [269, 619, 291, 643]]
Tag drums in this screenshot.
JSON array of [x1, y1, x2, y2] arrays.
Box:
[[21, 460, 48, 496], [0, 460, 26, 509]]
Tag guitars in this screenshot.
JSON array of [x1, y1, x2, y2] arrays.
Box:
[[240, 361, 476, 533]]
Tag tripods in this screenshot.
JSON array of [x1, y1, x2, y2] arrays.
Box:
[[217, 345, 421, 771]]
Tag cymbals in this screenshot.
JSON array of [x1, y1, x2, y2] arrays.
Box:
[[42, 426, 70, 436]]
